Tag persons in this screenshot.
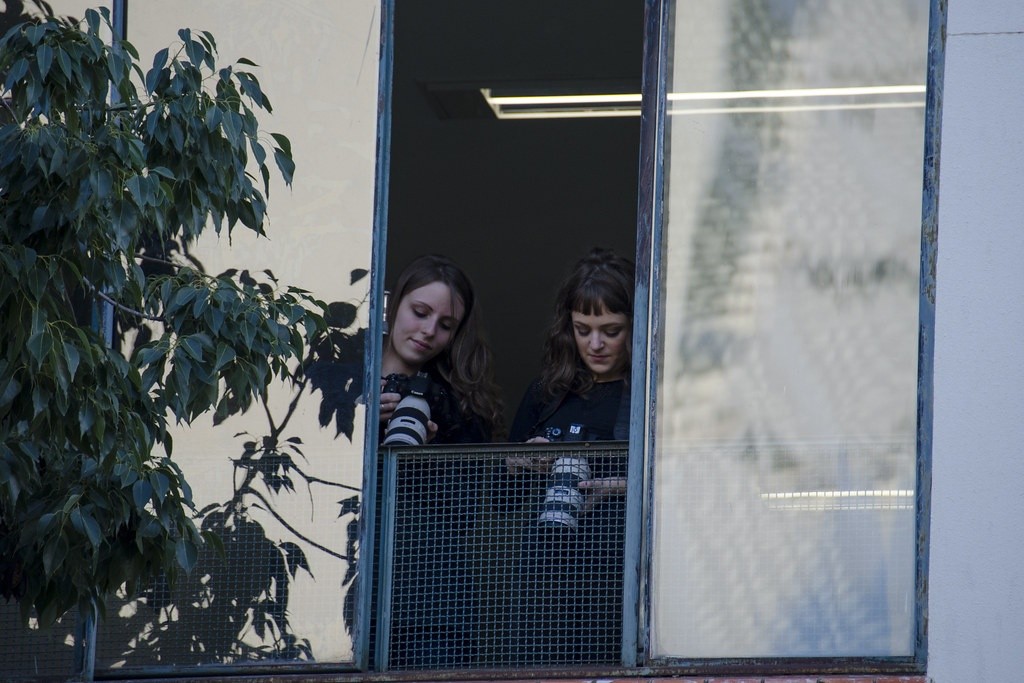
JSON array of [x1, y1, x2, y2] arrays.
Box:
[[492, 246, 639, 665], [367, 251, 508, 670]]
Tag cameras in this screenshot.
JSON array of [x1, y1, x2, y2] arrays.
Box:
[[381, 370, 447, 448], [539, 424, 598, 534]]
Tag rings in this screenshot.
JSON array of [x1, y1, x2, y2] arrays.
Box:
[[381, 404, 384, 412]]
